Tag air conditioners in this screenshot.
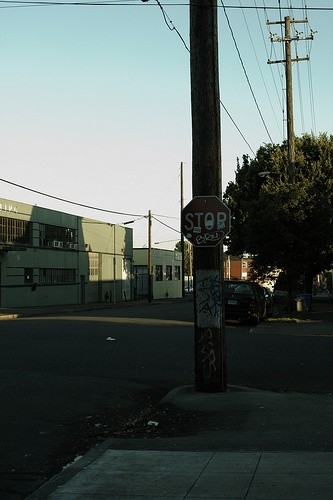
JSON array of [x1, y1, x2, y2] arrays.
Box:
[[68, 243, 75, 248], [53, 240, 63, 248]]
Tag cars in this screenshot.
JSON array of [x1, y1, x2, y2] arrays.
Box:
[[221, 280, 267, 327], [260, 285, 272, 315]]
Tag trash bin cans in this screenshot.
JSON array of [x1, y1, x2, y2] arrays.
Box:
[[295, 292, 313, 312]]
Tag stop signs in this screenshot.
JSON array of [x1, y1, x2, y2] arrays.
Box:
[[181, 196, 231, 248]]
